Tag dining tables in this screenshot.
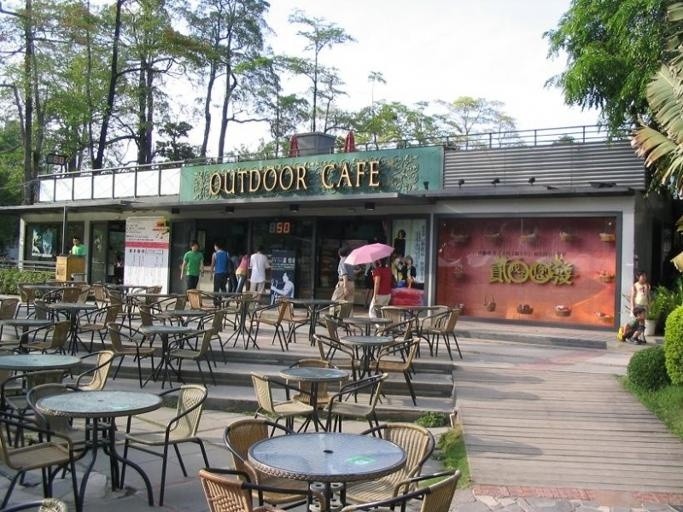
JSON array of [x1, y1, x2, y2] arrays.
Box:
[[621, 291, 666, 335]]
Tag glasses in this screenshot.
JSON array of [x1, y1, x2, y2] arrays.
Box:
[[226, 206, 234, 214], [171, 207, 180, 215], [289, 204, 299, 211], [365, 202, 375, 211]]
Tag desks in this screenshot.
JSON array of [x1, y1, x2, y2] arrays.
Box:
[[279, 367, 351, 433]]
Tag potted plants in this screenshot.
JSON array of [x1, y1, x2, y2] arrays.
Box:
[[621, 291, 666, 335]]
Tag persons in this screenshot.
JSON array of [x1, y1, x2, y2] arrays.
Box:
[[394, 229, 406, 257], [369, 256, 392, 337], [179, 240, 205, 290], [71, 234, 85, 256], [364, 264, 374, 309], [117, 255, 124, 268], [395, 254, 416, 280], [329, 247, 355, 320], [622, 307, 646, 344], [271, 272, 295, 305], [210, 240, 230, 293], [228, 255, 243, 293], [235, 254, 250, 293], [250, 245, 271, 291], [631, 270, 652, 343]]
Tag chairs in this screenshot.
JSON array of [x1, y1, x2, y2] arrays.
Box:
[[0, 282, 461, 406], [250, 374, 314, 440], [199, 468, 326, 512], [107, 263, 123, 284], [120, 384, 208, 505], [0, 412, 81, 512], [323, 372, 391, 438], [223, 420, 307, 509], [26, 383, 115, 498], [628, 337, 640, 345], [335, 422, 435, 508], [0, 499, 68, 511], [340, 469, 461, 512]]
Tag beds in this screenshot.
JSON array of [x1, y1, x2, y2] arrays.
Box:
[[343, 242, 396, 266]]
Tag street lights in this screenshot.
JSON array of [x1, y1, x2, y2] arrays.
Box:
[[617, 327, 624, 341]]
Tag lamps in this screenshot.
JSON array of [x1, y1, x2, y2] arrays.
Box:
[[226, 206, 234, 214], [0, 412, 81, 512], [171, 207, 180, 215], [26, 383, 115, 498], [199, 468, 326, 512], [289, 204, 299, 211], [0, 499, 68, 511], [365, 202, 375, 211], [335, 422, 435, 508], [107, 263, 123, 284], [223, 420, 307, 509], [120, 384, 208, 505], [340, 469, 461, 512]]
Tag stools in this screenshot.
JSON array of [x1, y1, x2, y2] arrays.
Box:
[[339, 278, 352, 281]]
